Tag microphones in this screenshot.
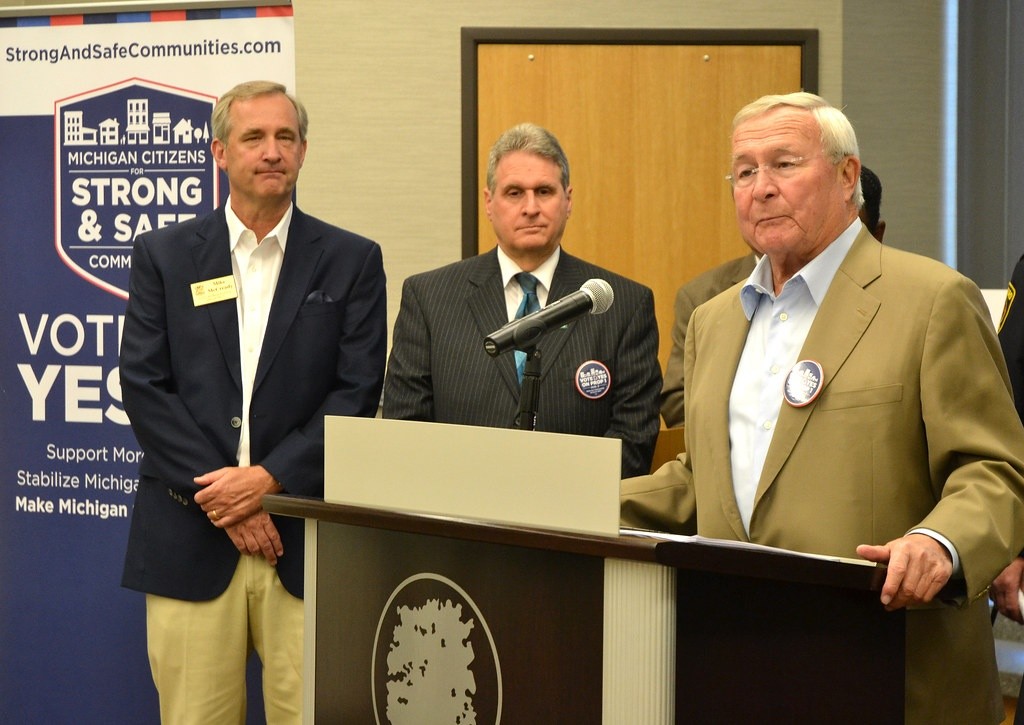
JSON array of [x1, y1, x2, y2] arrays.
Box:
[[484, 279, 614, 358]]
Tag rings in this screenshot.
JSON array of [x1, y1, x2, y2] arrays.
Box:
[[212, 510, 220, 520]]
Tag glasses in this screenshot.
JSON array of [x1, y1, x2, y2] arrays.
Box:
[[723, 150, 827, 189]]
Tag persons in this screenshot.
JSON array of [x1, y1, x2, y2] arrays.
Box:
[[660, 234, 762, 430], [988, 254, 1024, 628], [119, 79, 389, 725], [381, 123, 664, 477], [615, 90, 1024, 725]]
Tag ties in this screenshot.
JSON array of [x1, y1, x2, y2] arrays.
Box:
[[512, 271, 543, 387]]
